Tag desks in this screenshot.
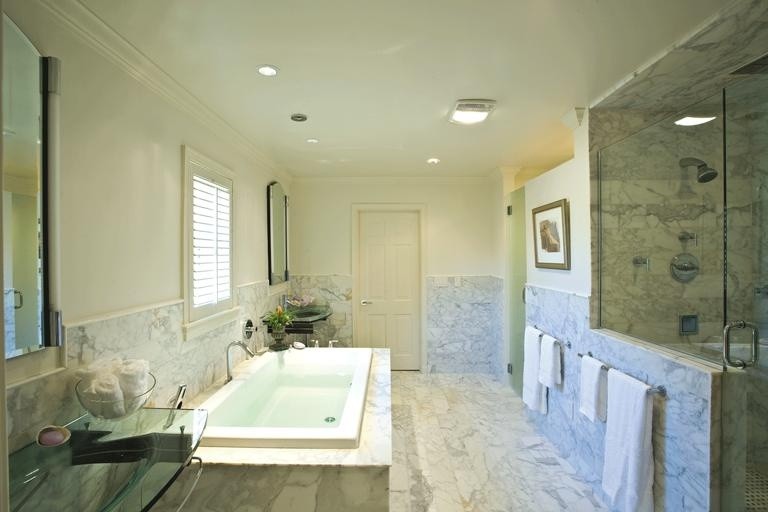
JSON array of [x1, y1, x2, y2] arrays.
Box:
[[6, 400, 209, 510]]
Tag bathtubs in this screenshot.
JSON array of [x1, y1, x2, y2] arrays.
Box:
[[690, 343, 767, 373], [163, 347, 374, 448]]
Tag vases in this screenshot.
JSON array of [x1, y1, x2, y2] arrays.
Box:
[[270, 324, 289, 352]]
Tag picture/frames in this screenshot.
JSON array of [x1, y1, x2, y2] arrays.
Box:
[[532, 198, 570, 272]]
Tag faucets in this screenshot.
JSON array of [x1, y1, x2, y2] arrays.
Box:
[[282, 294, 295, 311], [226, 340, 254, 379]]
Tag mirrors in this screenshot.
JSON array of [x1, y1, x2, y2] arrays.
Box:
[[265, 180, 290, 288], [1, 14, 65, 361]]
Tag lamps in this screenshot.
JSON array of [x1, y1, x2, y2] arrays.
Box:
[[446, 98, 497, 126], [674, 116, 716, 128]]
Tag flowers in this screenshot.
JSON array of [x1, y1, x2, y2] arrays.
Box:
[[262, 306, 297, 328]]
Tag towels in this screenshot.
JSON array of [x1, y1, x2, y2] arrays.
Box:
[[578, 354, 606, 423], [536, 335, 562, 390], [75, 356, 150, 418], [522, 326, 548, 414], [600, 365, 657, 511]]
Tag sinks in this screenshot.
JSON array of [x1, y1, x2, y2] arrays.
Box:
[[294, 309, 321, 318]]
